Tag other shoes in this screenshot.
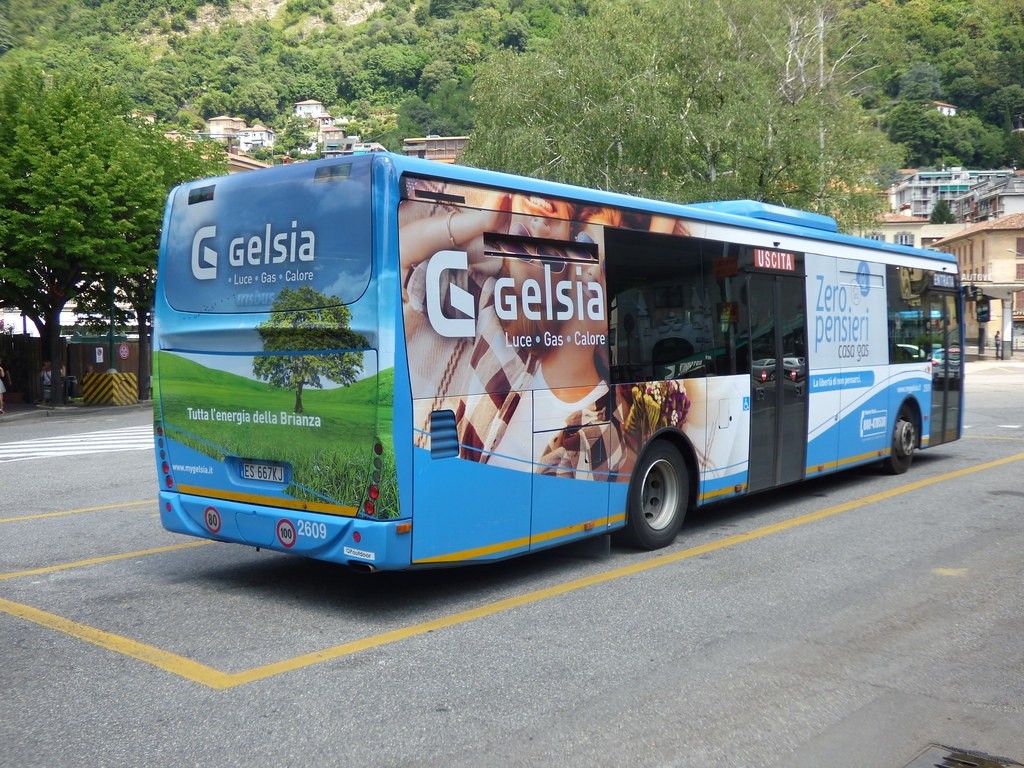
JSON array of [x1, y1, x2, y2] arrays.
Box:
[[0, 409, 5, 414]]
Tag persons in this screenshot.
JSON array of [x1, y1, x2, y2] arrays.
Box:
[[0, 367, 6, 413], [399, 176, 676, 483], [40, 360, 51, 389], [995, 331, 1001, 358]]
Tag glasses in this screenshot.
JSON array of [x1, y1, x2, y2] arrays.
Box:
[[574, 232, 598, 260], [509, 222, 568, 275]]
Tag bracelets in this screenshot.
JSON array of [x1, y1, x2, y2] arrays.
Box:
[[443, 210, 459, 251]]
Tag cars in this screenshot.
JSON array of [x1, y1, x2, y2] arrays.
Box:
[[948, 347, 960, 390], [933, 348, 945, 390], [752, 359, 776, 383], [784, 357, 805, 383]]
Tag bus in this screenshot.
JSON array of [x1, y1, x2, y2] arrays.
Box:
[[152, 152, 991, 572]]
